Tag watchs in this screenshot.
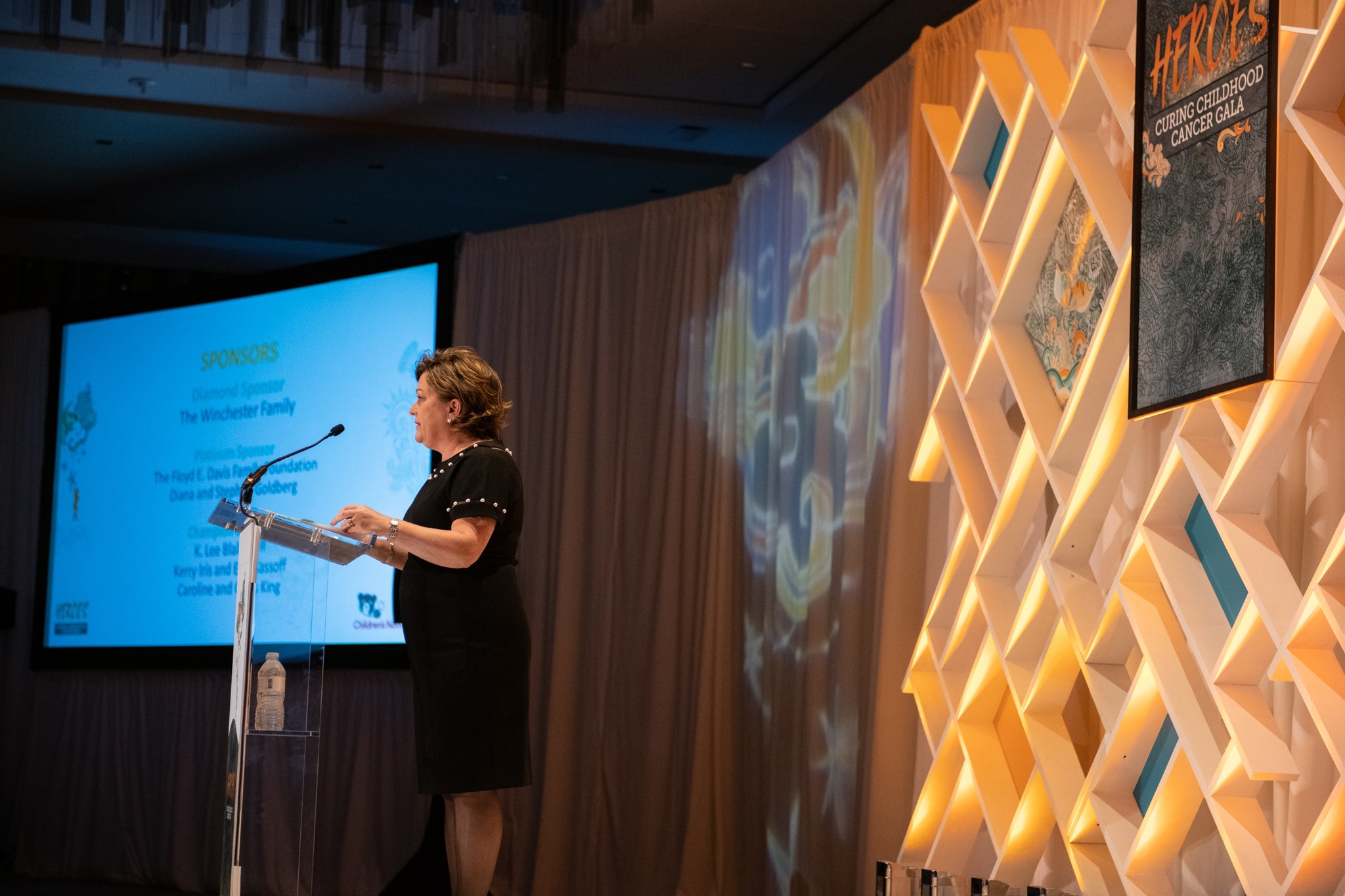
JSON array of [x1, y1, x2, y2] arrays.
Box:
[[387, 518, 399, 543]]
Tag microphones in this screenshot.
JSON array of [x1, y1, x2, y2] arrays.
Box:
[[240, 423, 345, 490]]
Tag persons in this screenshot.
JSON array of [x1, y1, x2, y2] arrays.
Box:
[[329, 346, 534, 896]]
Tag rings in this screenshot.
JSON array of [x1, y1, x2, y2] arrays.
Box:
[[347, 519, 354, 527]]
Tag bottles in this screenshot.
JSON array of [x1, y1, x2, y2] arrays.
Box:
[[255, 652, 286, 731]]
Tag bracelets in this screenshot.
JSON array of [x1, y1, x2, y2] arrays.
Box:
[[382, 542, 394, 565]]
